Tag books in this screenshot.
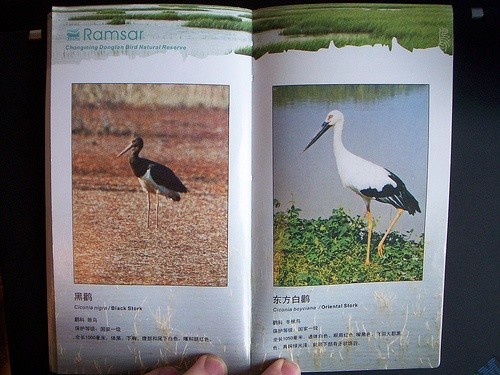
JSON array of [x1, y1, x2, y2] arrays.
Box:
[[46, 4, 454, 375]]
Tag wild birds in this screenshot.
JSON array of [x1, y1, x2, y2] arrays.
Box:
[[116, 137, 187, 228], [304, 110, 421, 265]]
